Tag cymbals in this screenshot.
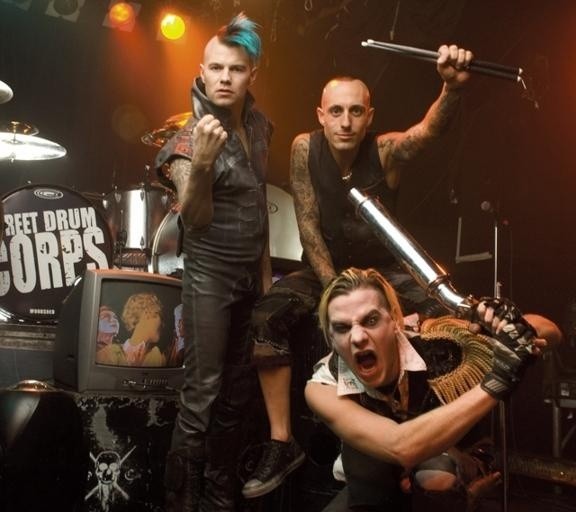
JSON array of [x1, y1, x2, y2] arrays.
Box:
[[1, 80, 12, 105], [0, 131, 67, 160], [142, 111, 192, 148], [0, 119, 40, 134]]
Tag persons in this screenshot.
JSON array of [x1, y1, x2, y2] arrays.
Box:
[[96, 304, 119, 348], [302, 267, 565, 510], [243, 44, 475, 498], [152, 9, 277, 511], [166, 304, 185, 366], [96, 293, 166, 367]]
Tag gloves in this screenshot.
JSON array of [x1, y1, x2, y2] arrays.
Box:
[[481, 324, 540, 398], [469, 295, 522, 333]]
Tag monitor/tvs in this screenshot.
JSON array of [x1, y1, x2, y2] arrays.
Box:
[[53, 268, 185, 395]]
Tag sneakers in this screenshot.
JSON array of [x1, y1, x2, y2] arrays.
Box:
[[241, 434, 307, 497]]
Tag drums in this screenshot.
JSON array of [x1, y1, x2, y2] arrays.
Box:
[[0, 183, 115, 322], [152, 182, 306, 284], [101, 182, 176, 265]]
